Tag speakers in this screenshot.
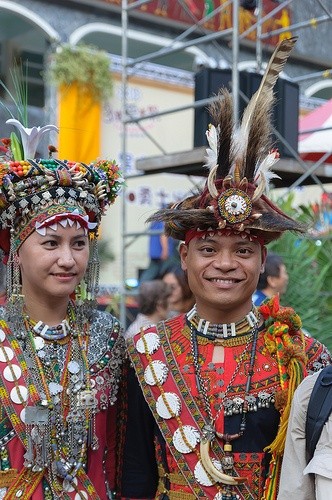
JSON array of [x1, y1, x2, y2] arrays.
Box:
[[194, 67, 232, 147], [239, 71, 264, 127], [271, 78, 300, 158]]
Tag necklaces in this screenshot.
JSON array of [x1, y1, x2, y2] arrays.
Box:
[[7, 296, 97, 500], [189, 311, 263, 472]]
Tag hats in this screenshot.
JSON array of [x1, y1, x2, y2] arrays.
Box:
[[145, 36, 308, 246], [0, 158, 127, 340]]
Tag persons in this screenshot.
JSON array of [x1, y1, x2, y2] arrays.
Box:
[[1, 191, 125, 500], [277, 361, 332, 500], [123, 255, 289, 340], [118, 188, 331, 500]]
